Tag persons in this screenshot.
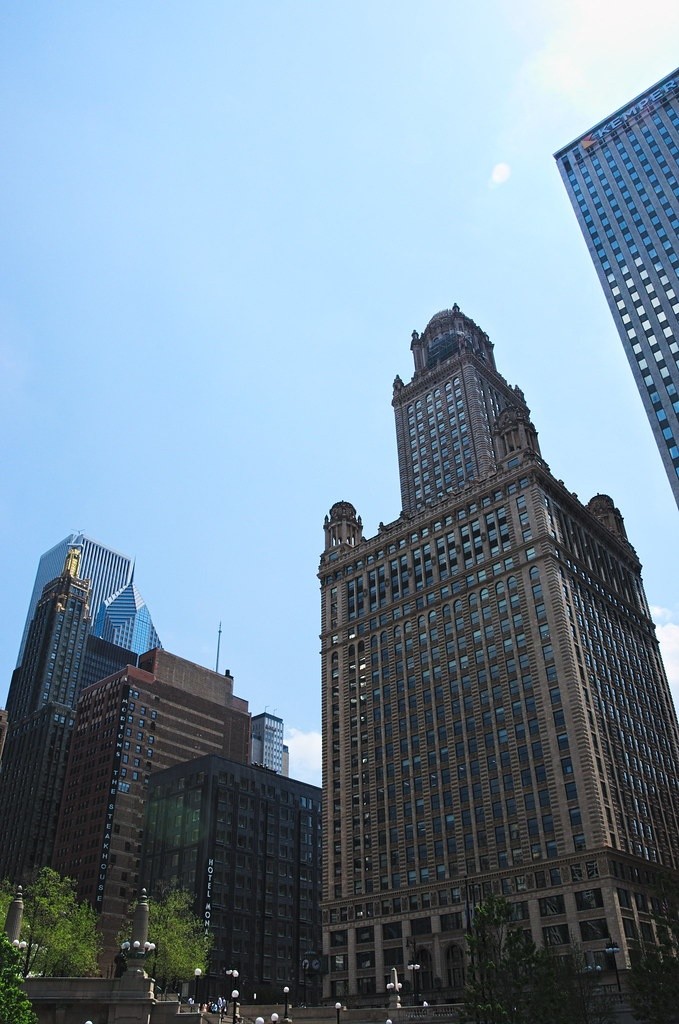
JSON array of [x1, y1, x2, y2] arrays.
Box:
[[188, 996, 194, 1004], [202, 995, 228, 1015], [423, 1000, 430, 1015]]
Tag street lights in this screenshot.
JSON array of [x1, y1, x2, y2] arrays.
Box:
[[284, 986, 290, 1019], [225, 969, 240, 1003], [231, 989, 240, 1024], [194, 967, 202, 1003], [604, 936, 623, 1004], [334, 1002, 341, 1024], [408, 959, 420, 1006]]
